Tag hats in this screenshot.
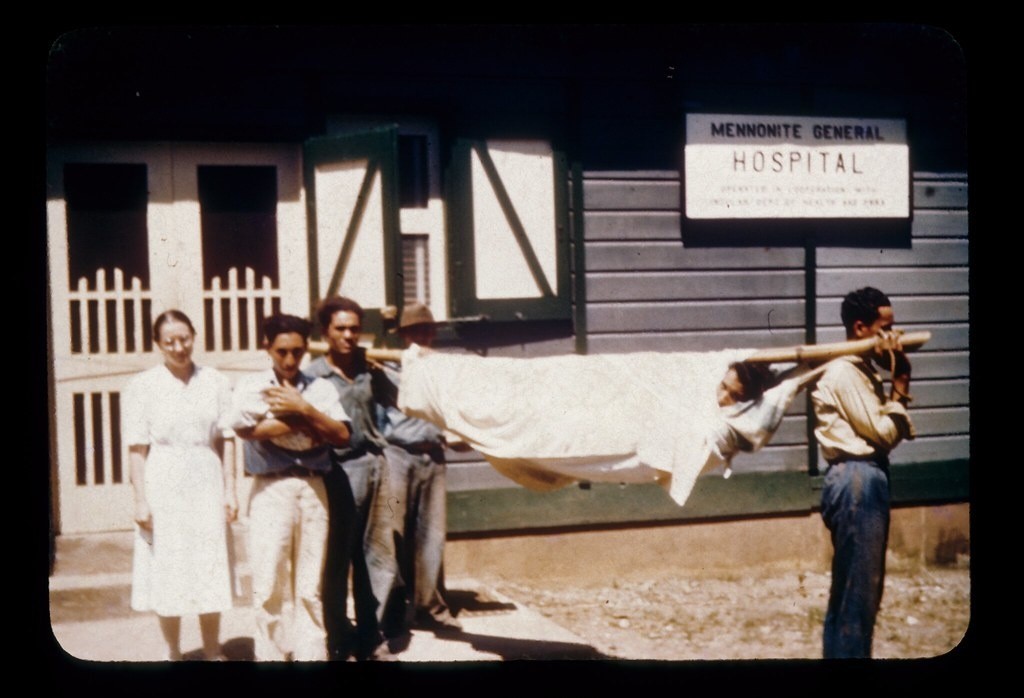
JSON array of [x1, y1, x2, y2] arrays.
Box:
[[388, 301, 448, 333]]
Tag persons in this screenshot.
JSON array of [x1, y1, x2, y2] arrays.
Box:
[[228, 295, 459, 662], [122, 310, 240, 662], [399, 355, 763, 484], [811, 285, 914, 660]]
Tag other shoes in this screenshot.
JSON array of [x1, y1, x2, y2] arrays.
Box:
[[434, 616, 466, 639]]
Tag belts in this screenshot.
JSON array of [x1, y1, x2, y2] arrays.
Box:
[[279, 464, 316, 476]]
